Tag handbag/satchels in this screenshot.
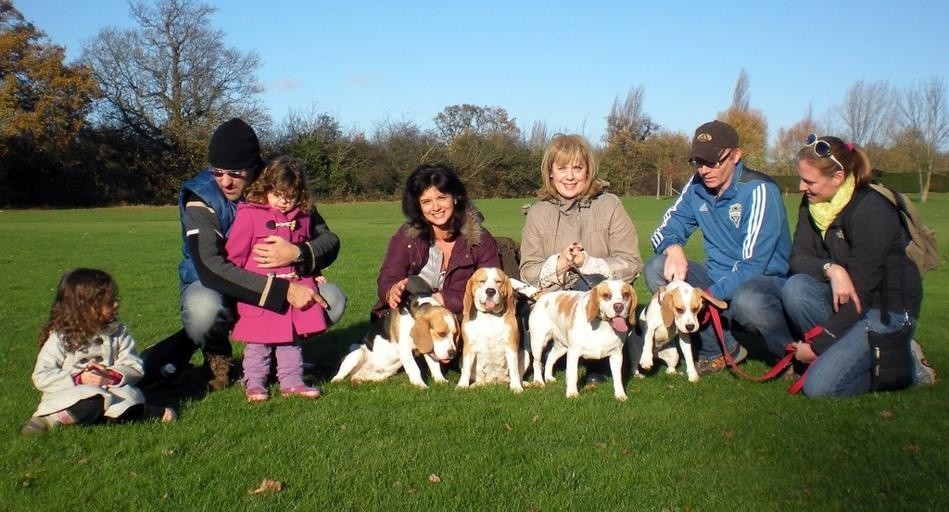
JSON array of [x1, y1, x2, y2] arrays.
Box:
[[866, 320, 913, 392], [495, 235, 520, 279]]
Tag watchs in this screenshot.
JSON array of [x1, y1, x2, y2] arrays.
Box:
[[823, 262, 832, 276], [294, 247, 304, 264]]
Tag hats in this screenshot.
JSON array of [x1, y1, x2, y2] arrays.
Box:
[[207, 117, 260, 169], [689, 120, 739, 164]]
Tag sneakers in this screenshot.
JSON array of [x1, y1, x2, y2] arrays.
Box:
[[303, 334, 338, 365], [910, 340, 936, 384], [202, 350, 233, 391], [773, 364, 800, 382], [695, 342, 747, 374]]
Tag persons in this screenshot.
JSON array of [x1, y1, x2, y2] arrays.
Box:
[[222, 155, 328, 401], [176, 116, 349, 392], [781, 129, 938, 398], [518, 133, 644, 385], [360, 161, 505, 352], [643, 119, 793, 378], [22, 266, 179, 434]]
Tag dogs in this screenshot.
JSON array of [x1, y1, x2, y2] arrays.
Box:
[[331, 297, 460, 389], [454, 267, 533, 393], [626, 281, 728, 382], [509, 278, 638, 401]]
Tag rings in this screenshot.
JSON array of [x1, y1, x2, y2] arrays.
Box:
[[264, 257, 267, 263]]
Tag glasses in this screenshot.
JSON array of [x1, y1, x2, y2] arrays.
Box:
[[805, 133, 844, 170], [208, 165, 244, 178], [689, 152, 732, 171]]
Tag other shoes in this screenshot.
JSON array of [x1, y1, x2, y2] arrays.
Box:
[[581, 359, 609, 384], [422, 358, 449, 379], [246, 386, 268, 400], [280, 385, 320, 398], [161, 407, 177, 422], [21, 416, 49, 437]]
